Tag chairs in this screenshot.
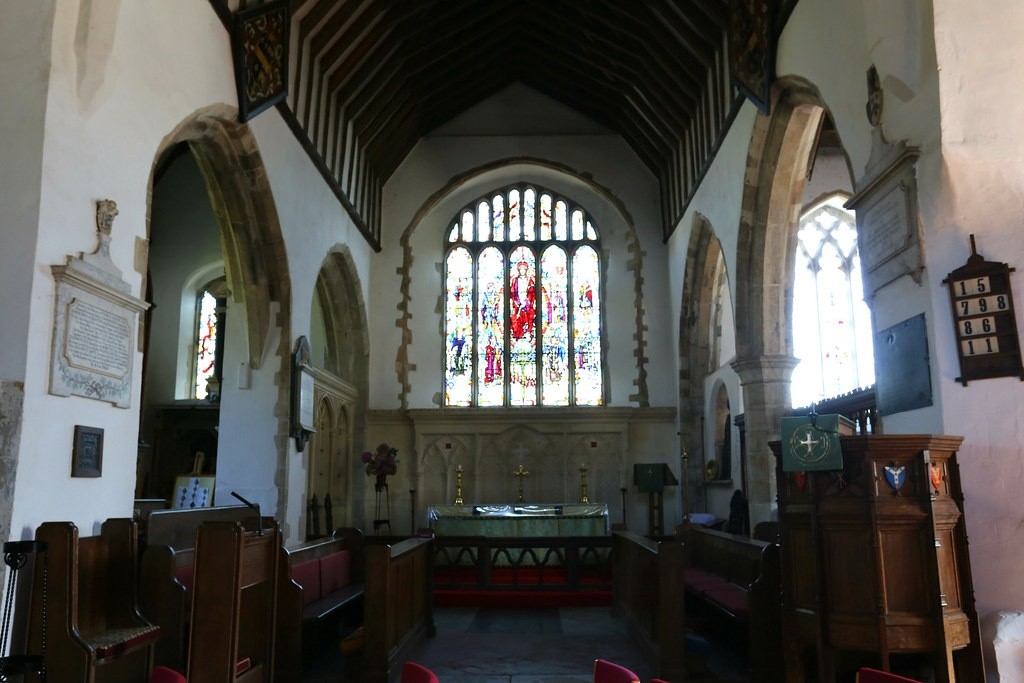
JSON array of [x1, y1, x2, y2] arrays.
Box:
[[400, 662, 438, 683], [151, 666, 185, 683], [593, 659, 640, 683]]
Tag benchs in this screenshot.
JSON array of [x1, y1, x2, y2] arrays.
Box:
[[676, 528, 773, 622], [291, 539, 365, 623], [85, 624, 161, 664]]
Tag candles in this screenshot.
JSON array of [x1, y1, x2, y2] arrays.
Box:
[[581, 462, 584, 469], [458, 464, 461, 471], [519, 464, 523, 472]]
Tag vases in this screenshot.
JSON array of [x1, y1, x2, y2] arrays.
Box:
[[376, 474, 386, 484]]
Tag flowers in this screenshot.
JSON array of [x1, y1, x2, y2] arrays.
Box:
[[362, 443, 399, 475]]
